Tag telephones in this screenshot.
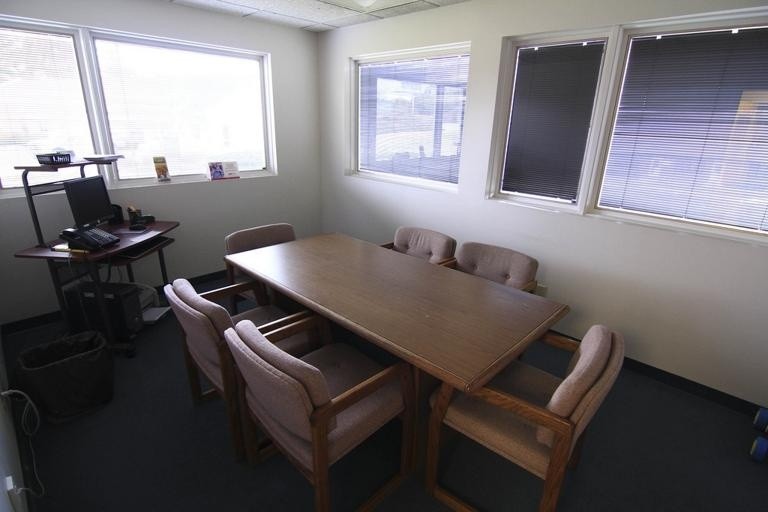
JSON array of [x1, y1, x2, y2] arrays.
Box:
[[58, 222, 120, 252]]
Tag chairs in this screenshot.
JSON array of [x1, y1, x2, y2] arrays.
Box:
[[423, 322, 626, 512], [380, 227, 455, 268], [433, 240, 540, 295], [224, 223, 295, 316], [159, 276, 319, 462], [226, 313, 416, 512]]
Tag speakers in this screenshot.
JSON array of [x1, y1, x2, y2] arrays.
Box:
[[109, 204, 124, 225]]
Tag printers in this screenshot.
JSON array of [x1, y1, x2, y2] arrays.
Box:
[[130, 282, 171, 326]]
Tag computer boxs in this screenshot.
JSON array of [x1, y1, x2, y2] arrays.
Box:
[[80, 283, 144, 339]]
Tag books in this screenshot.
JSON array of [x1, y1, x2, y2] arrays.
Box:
[[51, 243, 89, 253]]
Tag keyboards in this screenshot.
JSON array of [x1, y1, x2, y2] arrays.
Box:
[[119, 236, 169, 259]]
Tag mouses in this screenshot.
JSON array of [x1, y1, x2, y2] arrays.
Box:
[[129, 224, 145, 230]]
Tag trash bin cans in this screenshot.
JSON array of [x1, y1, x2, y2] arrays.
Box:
[[17, 329, 114, 417]]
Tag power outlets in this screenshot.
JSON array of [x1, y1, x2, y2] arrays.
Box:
[[5, 473, 25, 512]]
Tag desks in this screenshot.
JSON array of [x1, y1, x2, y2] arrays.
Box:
[[222, 232, 571, 512], [11, 159, 180, 359]]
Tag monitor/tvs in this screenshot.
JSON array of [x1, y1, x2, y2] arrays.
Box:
[[63, 175, 115, 227]]
